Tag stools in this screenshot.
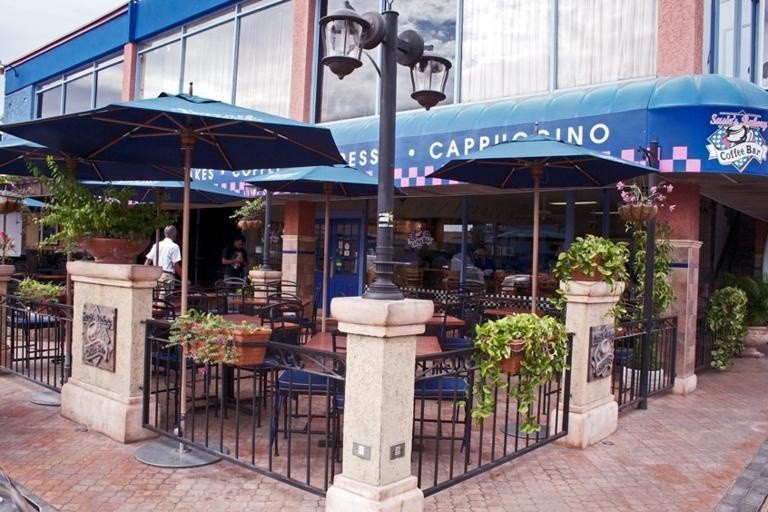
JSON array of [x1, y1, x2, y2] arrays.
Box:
[[7, 313, 65, 369]]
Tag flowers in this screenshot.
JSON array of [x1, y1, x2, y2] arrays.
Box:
[[616, 180, 676, 212]]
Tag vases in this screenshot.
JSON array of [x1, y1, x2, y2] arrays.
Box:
[[617, 204, 658, 223]]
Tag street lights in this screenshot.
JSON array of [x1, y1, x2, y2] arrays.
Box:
[[319, 0, 452, 300]]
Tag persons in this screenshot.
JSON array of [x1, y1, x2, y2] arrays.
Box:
[[142, 225, 192, 312], [449, 241, 474, 270], [472, 245, 497, 269], [222, 236, 251, 286]]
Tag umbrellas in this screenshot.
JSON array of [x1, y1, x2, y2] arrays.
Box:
[[0, 185, 59, 259], [1, 82, 351, 451], [423, 120, 660, 424], [78, 178, 257, 267], [244, 164, 410, 334], [0, 134, 194, 387]]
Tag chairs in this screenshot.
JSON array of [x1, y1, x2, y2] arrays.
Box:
[[269, 279, 490, 464], [150, 271, 317, 428]]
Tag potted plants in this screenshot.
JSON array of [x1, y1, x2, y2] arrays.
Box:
[[0, 155, 181, 263], [736, 308, 768, 358], [228, 195, 268, 230], [549, 233, 630, 334], [621, 220, 677, 396], [476, 311, 572, 435], [19, 276, 69, 315]]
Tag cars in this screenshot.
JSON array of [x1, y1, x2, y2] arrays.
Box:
[[338, 227, 409, 273]]
